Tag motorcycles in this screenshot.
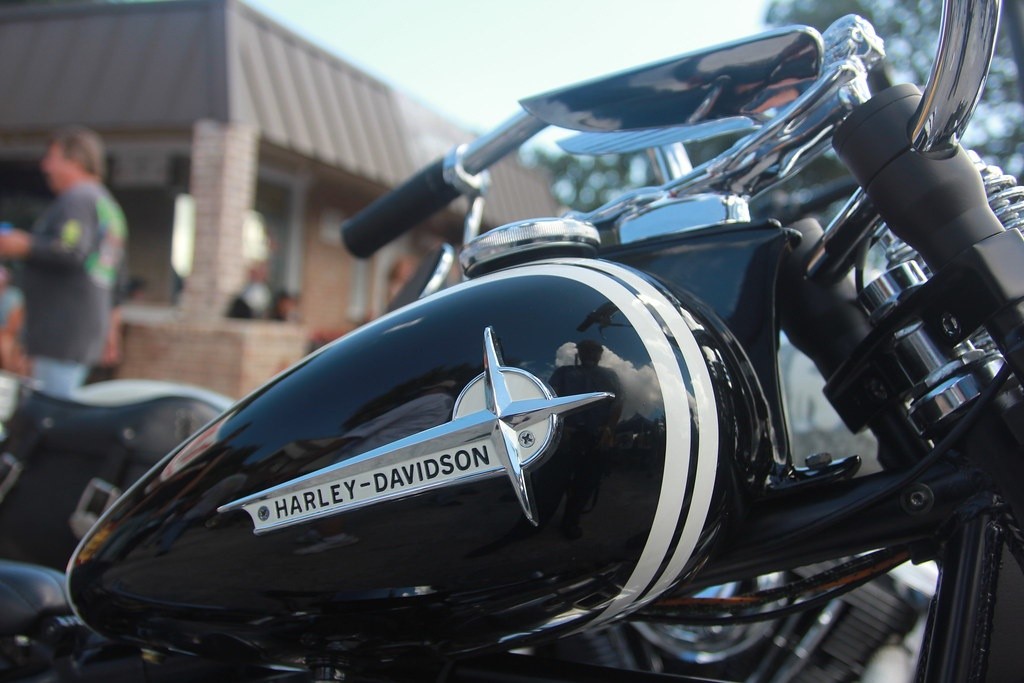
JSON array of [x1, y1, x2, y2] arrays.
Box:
[[1, 0, 1023, 683]]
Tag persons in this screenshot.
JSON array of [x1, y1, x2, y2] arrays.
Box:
[[154, 463, 256, 557], [519, 340, 625, 537], [0, 126, 133, 388], [278, 379, 456, 556], [229, 264, 293, 322]]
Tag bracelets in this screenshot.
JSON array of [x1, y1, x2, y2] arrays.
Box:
[[606, 426, 611, 438]]
